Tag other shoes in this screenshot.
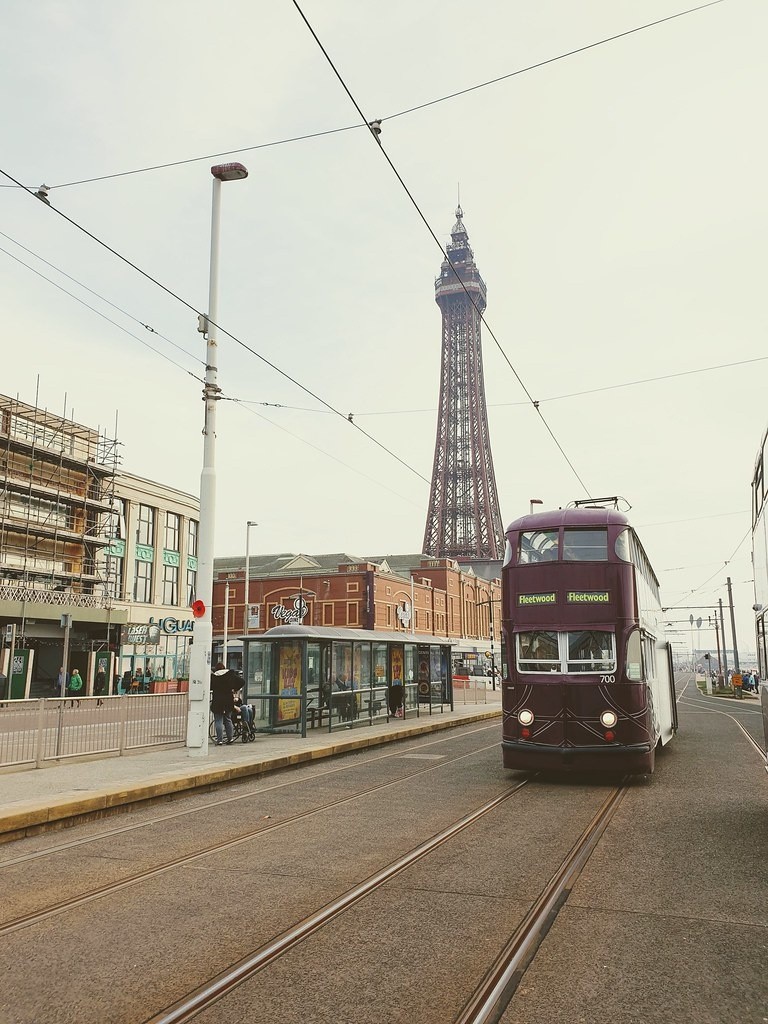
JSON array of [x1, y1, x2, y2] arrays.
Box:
[[216, 740, 233, 746]]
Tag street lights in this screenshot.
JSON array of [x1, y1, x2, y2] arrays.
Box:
[[186, 160, 248, 756], [241, 520, 259, 636]]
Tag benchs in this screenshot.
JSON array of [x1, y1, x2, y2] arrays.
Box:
[[364, 696, 388, 715], [307, 704, 338, 729]]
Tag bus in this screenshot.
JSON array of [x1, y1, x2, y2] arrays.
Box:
[[490, 495, 679, 787]]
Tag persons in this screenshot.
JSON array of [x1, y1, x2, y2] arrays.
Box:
[[94, 667, 105, 708], [146, 667, 151, 677], [698, 668, 760, 694], [66, 669, 83, 708], [136, 667, 142, 677], [322, 674, 350, 722], [334, 673, 357, 719], [232, 670, 257, 733], [520, 645, 560, 671], [210, 663, 245, 745], [384, 679, 404, 718], [55, 667, 70, 707]]
